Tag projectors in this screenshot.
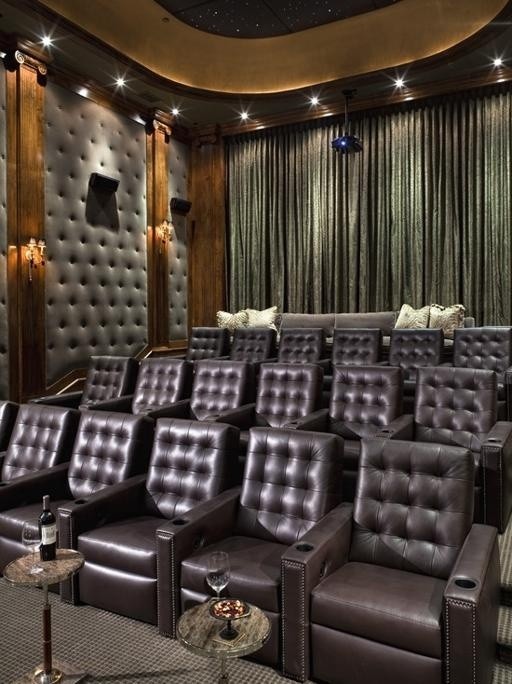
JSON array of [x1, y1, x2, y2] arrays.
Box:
[[332, 137, 362, 152]]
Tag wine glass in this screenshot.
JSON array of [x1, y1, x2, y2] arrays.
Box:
[[21, 519, 43, 576], [204, 551, 231, 601]]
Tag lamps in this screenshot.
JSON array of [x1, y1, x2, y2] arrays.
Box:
[[20, 232, 50, 284], [153, 217, 180, 253]]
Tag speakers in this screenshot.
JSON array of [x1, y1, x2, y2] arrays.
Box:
[[171, 198, 192, 212], [89, 173, 120, 192]]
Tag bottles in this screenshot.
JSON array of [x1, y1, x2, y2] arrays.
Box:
[[38, 495, 58, 562]]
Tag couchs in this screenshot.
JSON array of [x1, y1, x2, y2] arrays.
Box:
[[0, 297, 512, 536], [2, 400, 500, 682]]
